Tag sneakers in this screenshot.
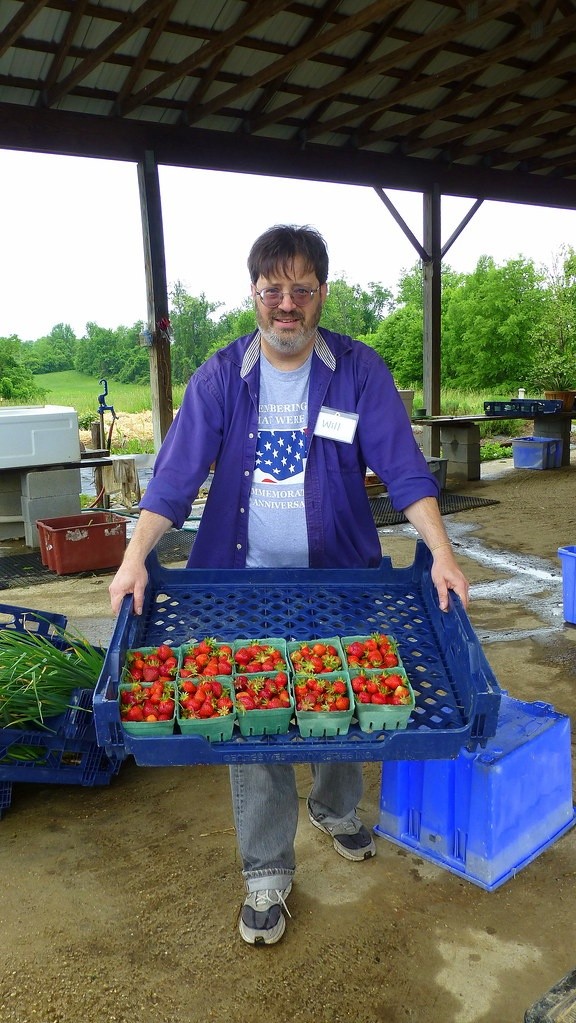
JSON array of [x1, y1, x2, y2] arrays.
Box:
[[238, 880, 295, 948], [306, 802, 377, 861]]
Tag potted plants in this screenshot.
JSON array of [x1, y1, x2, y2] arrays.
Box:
[[536, 377, 576, 412]]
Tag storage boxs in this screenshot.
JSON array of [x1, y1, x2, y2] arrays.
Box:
[[0, 604, 67, 638], [35, 512, 131, 575], [511, 436, 563, 470], [93, 539, 502, 767], [373, 689, 576, 891], [425, 456, 449, 490], [0, 627, 122, 787], [0, 405, 81, 469], [558, 545, 576, 624]]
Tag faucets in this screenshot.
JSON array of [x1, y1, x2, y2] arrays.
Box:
[[97, 378, 119, 420]]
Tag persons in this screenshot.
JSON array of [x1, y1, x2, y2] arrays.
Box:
[[109, 229, 468, 944]]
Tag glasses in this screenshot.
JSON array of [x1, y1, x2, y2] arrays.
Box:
[[253, 281, 328, 309]]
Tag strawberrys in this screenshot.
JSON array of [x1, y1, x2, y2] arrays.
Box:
[[119, 635, 412, 723]]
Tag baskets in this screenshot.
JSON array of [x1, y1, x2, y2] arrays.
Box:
[[88, 544, 506, 770], [482, 398, 564, 419]]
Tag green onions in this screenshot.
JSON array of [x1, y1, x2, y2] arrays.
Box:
[[0, 612, 104, 765]]
